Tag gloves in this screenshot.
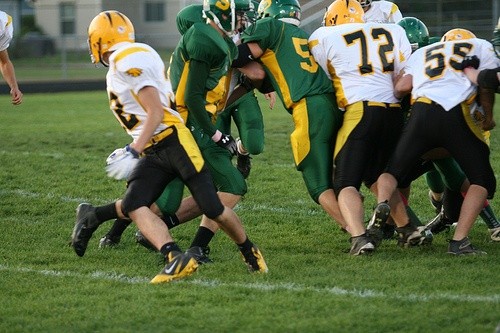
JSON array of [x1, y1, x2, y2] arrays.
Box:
[[215, 133, 238, 156], [104, 143, 142, 181]]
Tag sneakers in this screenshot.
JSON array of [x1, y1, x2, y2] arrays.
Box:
[[148, 250, 199, 285], [135, 229, 158, 253], [98, 234, 120, 250], [184, 245, 210, 264], [338, 199, 500, 257], [70, 202, 101, 257], [240, 243, 269, 275]]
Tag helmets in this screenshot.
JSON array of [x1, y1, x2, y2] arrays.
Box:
[[201, 0, 255, 36], [87, 10, 136, 69], [395, 16, 430, 54], [257, 0, 302, 27], [439, 28, 477, 42], [322, 0, 366, 27]]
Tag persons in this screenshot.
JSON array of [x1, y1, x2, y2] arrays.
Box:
[[229, 0, 500, 256], [87, 10, 270, 283], [0, 11, 23, 105], [73, 0, 275, 257]]
